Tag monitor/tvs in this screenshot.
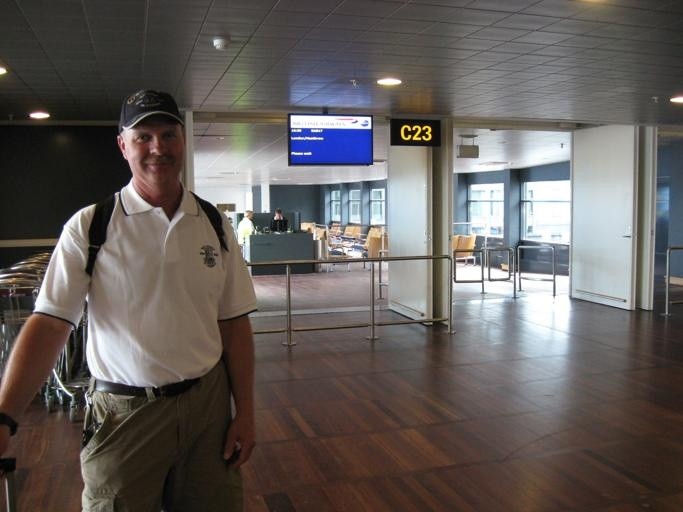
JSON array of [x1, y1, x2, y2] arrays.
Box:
[[287, 113, 373, 166]]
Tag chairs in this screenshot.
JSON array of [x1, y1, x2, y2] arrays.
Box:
[[300, 216, 388, 273], [454, 235, 476, 265]]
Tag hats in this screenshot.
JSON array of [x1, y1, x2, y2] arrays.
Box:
[[118, 88, 184, 133]]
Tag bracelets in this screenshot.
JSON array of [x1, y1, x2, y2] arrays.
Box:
[[0, 412, 18, 436]]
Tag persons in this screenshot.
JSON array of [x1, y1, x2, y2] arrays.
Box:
[[238, 211, 255, 244], [270, 208, 288, 232], [0, 89, 258, 511]]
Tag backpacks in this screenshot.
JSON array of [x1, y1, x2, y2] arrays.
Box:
[[60, 190, 230, 385]]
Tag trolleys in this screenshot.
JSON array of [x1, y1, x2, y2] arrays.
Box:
[[0, 247, 93, 432]]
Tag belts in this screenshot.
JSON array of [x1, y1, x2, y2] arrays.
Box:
[[94, 377, 201, 398]]
